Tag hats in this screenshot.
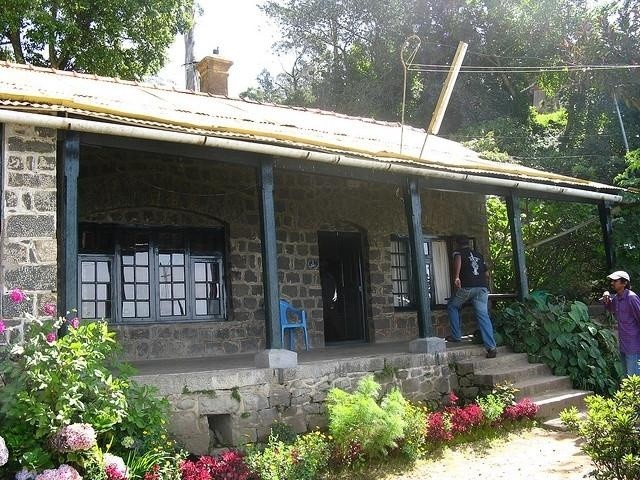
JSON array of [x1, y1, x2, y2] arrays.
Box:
[[607, 270, 631, 282]]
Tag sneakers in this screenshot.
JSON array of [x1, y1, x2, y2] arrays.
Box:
[[444, 336, 457, 342], [487, 347, 496, 358]]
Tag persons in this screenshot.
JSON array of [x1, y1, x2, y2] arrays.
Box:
[[445, 233, 496, 358], [598, 271, 639, 377]]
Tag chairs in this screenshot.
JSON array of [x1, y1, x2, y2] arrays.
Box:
[[279, 300, 310, 353]]
